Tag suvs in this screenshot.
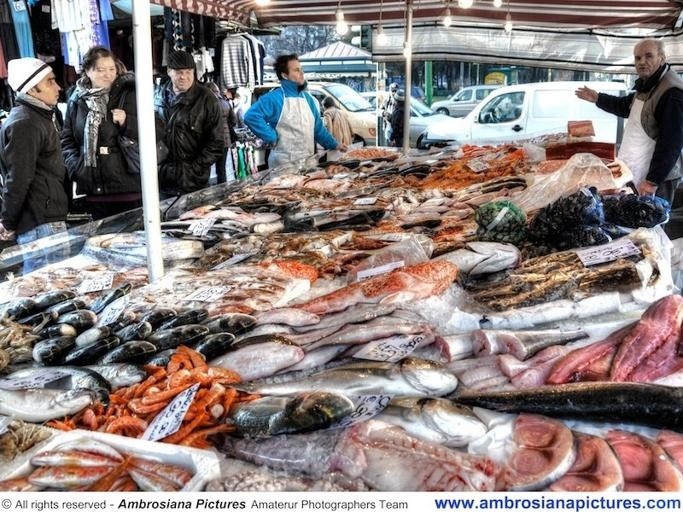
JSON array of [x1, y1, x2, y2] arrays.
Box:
[[432, 86, 501, 117]]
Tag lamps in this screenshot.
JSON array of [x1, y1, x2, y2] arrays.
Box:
[[335, 0, 349, 35], [493, 0, 503, 8], [376, 0, 389, 48], [443, 8, 452, 27], [458, 0, 474, 9], [505, 0, 513, 32]]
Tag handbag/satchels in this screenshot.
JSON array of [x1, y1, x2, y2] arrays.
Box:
[[117, 89, 169, 175]]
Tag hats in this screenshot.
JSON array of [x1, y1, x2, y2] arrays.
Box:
[[6, 57, 53, 95], [166, 50, 194, 69], [393, 89, 404, 102]]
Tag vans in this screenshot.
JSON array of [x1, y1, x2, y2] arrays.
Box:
[[467, 82, 627, 144], [253, 84, 385, 142], [361, 90, 441, 148]]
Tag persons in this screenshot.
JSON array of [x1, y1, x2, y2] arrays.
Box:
[[153, 50, 224, 221], [572, 40, 682, 210], [0, 56, 74, 276], [204, 77, 408, 185], [59, 46, 144, 235], [242, 54, 348, 179]]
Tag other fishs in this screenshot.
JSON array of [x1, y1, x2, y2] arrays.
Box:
[[0, 132, 683, 493]]
